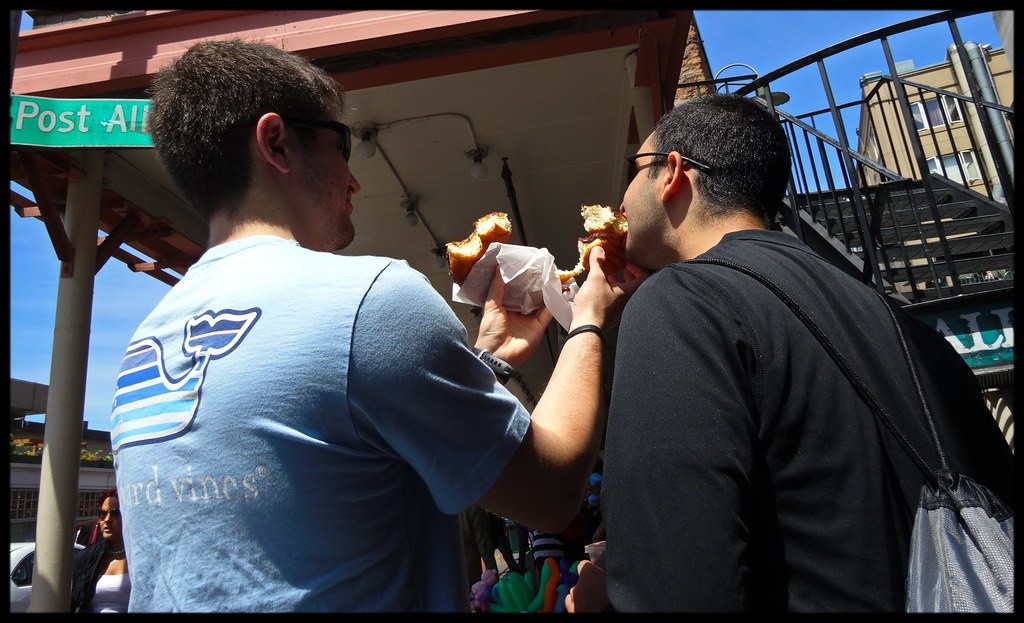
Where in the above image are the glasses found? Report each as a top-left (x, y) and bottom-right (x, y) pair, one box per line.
(280, 114), (352, 165)
(97, 508), (121, 519)
(626, 152), (712, 184)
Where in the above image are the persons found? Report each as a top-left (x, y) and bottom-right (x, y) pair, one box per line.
(65, 486), (130, 613)
(516, 524), (528, 574)
(463, 505), (524, 583)
(109, 38), (664, 613)
(526, 526), (564, 588)
(563, 94), (1015, 615)
(570, 471), (605, 546)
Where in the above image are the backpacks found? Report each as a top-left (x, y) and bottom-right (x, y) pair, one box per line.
(682, 254), (1014, 614)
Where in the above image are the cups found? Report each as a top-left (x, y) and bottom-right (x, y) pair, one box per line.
(584, 541), (606, 573)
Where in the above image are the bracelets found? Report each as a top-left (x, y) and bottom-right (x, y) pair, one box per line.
(563, 323), (603, 345)
(470, 344), (514, 386)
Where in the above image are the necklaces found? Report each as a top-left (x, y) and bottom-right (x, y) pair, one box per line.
(107, 544), (124, 554)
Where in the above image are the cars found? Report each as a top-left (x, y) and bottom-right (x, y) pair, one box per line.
(10, 518), (100, 614)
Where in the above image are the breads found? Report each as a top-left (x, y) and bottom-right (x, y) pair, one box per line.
(447, 204), (628, 287)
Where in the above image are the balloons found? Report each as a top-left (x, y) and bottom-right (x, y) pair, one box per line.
(489, 556), (579, 613)
